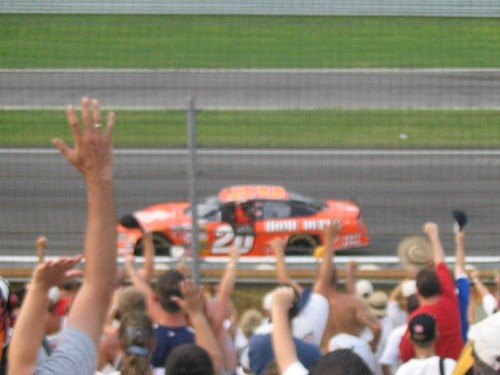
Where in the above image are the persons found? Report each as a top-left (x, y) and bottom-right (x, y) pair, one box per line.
(36, 97), (116, 375)
(456, 231), (500, 375)
(395, 314), (457, 375)
(398, 222), (464, 361)
(239, 217), (418, 375)
(0, 227), (247, 375)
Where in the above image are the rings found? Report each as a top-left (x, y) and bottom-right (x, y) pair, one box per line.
(94, 124), (102, 128)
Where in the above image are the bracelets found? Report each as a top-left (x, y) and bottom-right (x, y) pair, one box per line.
(474, 279), (480, 283)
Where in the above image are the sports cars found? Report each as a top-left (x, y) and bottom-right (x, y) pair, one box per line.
(117, 184), (370, 258)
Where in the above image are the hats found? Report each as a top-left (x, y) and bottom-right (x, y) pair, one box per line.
(114, 288), (147, 318)
(355, 279), (373, 299)
(408, 314), (437, 335)
(453, 210), (467, 234)
(152, 270), (183, 298)
(368, 291), (388, 317)
(399, 236), (433, 274)
